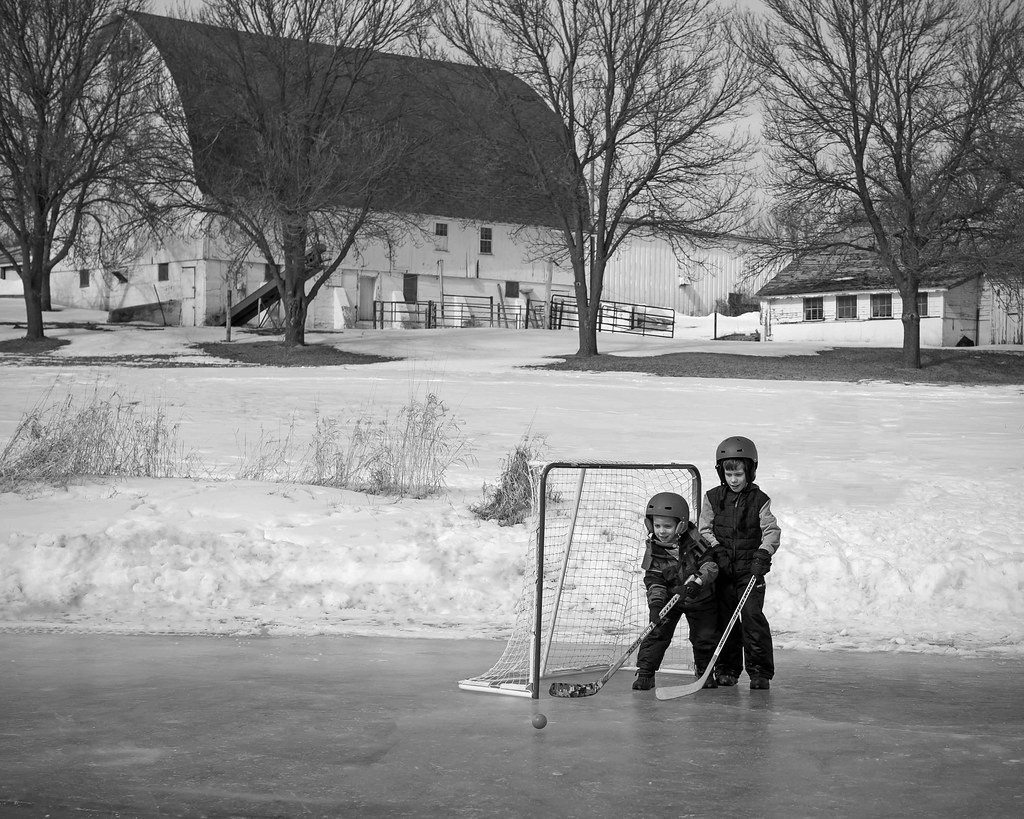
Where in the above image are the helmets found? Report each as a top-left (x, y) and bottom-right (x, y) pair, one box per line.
(644, 492), (690, 535)
(714, 436), (758, 482)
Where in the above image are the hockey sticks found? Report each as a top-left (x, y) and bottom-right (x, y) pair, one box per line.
(549, 573), (697, 697)
(654, 574), (757, 701)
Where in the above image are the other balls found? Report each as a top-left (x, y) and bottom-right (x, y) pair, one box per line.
(532, 714), (547, 729)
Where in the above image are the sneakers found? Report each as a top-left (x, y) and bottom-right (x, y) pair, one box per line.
(632, 669), (655, 689)
(694, 663), (718, 688)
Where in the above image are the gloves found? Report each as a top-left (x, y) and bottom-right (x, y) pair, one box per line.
(648, 602), (669, 629)
(712, 545), (733, 575)
(670, 581), (702, 610)
(750, 552), (769, 585)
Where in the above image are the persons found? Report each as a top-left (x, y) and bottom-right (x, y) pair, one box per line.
(698, 436), (781, 689)
(632, 492), (719, 690)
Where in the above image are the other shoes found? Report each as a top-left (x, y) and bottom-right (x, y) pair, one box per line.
(749, 674), (769, 689)
(715, 671), (738, 686)
